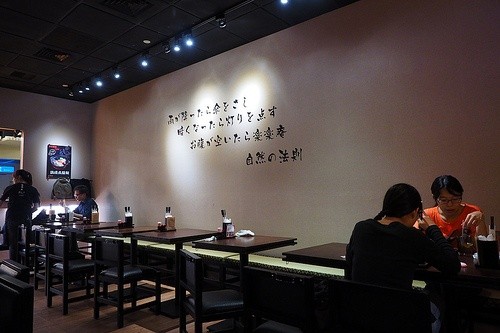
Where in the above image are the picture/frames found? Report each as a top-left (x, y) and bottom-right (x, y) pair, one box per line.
(44, 144), (72, 180)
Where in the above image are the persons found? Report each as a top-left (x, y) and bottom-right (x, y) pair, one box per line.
(343, 183), (461, 333)
(71, 184), (98, 221)
(414, 175), (488, 253)
(0, 169), (40, 250)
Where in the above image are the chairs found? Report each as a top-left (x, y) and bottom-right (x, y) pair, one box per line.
(15, 223), (448, 333)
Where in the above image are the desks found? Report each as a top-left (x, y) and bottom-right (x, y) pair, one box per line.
(90, 226), (159, 312)
(130, 228), (219, 320)
(416, 265), (500, 291)
(190, 234), (298, 333)
(34, 223), (119, 292)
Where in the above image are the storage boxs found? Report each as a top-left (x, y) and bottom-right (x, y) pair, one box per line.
(91, 213), (99, 222)
(83, 225), (100, 228)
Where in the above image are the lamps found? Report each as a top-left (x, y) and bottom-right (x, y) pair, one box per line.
(67, 13), (227, 97)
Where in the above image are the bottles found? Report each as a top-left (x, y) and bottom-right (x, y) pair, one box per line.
(118, 220), (121, 227)
(158, 222), (161, 230)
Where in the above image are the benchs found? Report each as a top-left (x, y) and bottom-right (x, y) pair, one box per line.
(122, 236), (161, 247)
(151, 245), (201, 255)
(226, 254), (348, 281)
(101, 236), (125, 241)
(461, 296), (500, 333)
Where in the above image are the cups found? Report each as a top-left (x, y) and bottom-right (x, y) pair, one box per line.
(49, 210), (55, 220)
(167, 217), (175, 229)
(460, 225), (474, 248)
(477, 239), (499, 269)
(125, 216), (132, 226)
(223, 223), (235, 238)
(92, 212), (99, 222)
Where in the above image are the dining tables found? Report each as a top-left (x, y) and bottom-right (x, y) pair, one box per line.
(280, 242), (349, 269)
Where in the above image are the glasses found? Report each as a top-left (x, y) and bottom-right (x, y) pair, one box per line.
(73, 193), (84, 197)
(436, 198), (462, 204)
